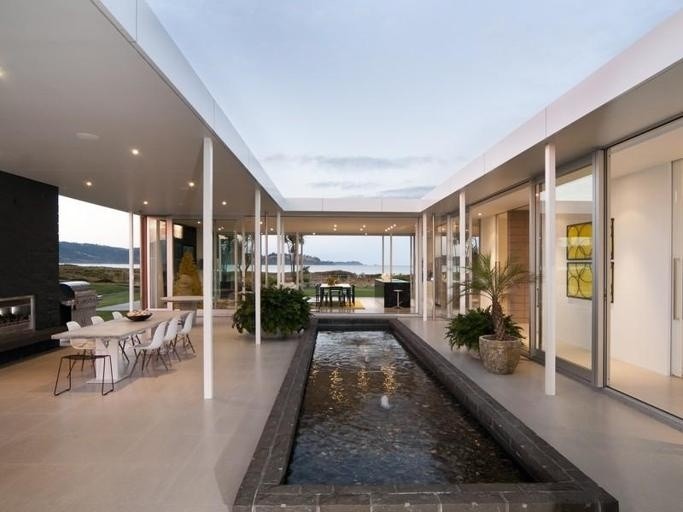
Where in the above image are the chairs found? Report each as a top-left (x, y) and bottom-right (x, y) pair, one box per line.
(315, 282), (356, 306)
(53, 309), (195, 374)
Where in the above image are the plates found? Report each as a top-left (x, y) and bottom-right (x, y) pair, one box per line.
(122, 312), (153, 321)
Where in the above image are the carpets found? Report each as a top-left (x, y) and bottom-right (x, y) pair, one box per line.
(307, 300), (365, 310)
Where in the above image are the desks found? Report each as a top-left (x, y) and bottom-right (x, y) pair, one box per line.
(319, 283), (351, 308)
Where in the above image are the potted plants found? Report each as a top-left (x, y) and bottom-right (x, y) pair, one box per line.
(443, 251), (544, 375)
(230, 285), (314, 340)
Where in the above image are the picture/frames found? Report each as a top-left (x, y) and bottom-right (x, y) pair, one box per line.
(566, 262), (614, 303)
(565, 217), (615, 260)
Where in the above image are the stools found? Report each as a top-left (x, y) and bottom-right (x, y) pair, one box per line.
(53, 354), (115, 397)
(392, 289), (405, 310)
